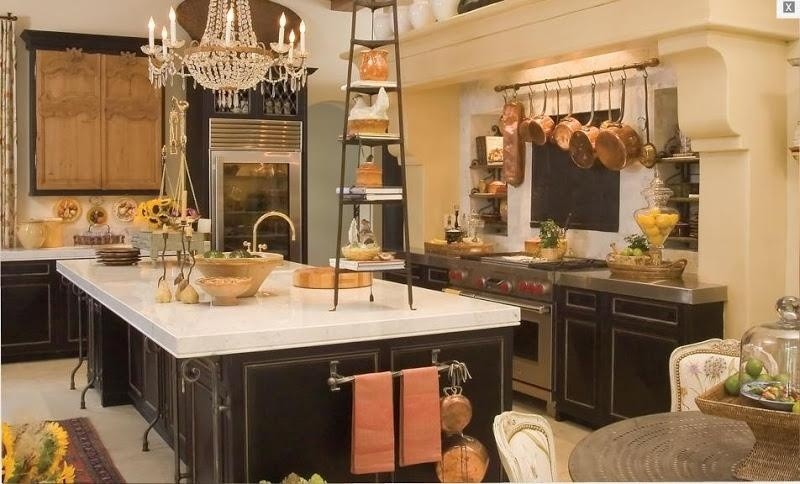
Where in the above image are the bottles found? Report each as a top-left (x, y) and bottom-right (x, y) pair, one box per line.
(479, 180), (486, 194)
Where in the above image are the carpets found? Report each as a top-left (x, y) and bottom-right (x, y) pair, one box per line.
(1, 417), (125, 484)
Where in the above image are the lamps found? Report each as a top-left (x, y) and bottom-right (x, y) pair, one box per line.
(140, 0), (310, 108)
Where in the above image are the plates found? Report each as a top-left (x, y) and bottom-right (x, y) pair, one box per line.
(739, 381), (797, 412)
(94, 248), (141, 268)
(112, 197), (138, 222)
(53, 198), (82, 222)
(86, 205), (109, 227)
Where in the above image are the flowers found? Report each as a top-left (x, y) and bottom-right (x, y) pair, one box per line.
(1, 421), (76, 484)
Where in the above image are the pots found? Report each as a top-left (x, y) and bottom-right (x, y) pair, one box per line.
(432, 434), (491, 482)
(517, 79), (645, 171)
(437, 385), (472, 436)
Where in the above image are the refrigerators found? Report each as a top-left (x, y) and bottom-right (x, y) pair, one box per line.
(208, 118), (304, 263)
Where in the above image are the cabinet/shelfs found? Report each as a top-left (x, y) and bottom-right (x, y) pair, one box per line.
(382, 263), (449, 291)
(556, 285), (723, 427)
(1, 260), (87, 359)
(329, 0), (417, 311)
(655, 154), (699, 242)
(470, 161), (507, 223)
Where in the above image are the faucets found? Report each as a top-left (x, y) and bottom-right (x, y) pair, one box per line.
(250, 210), (296, 252)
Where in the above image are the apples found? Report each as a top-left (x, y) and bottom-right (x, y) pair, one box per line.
(620, 249), (643, 256)
(724, 358), (791, 395)
(793, 399), (800, 413)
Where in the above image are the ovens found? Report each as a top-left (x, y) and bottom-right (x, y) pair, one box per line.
(445, 285), (558, 407)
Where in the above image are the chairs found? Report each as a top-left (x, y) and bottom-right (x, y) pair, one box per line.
(669, 338), (779, 412)
(492, 411), (556, 482)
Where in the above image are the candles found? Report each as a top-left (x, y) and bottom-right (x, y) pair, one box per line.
(163, 224), (168, 233)
(454, 200), (460, 209)
(186, 227), (192, 237)
(181, 189), (188, 221)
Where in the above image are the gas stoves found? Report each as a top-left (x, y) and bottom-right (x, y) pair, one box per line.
(447, 255), (610, 302)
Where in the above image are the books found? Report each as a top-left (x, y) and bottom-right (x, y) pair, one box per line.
(329, 258), (405, 272)
(336, 185), (403, 203)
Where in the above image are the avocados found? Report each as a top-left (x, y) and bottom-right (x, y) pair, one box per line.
(204, 250), (262, 259)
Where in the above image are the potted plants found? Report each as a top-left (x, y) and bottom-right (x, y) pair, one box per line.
(538, 218), (563, 260)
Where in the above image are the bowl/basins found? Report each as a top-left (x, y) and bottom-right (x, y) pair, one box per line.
(190, 252), (287, 302)
(340, 245), (382, 263)
(348, 118), (389, 135)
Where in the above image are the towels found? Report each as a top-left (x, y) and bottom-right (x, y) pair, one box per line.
(399, 366), (442, 468)
(351, 371), (395, 475)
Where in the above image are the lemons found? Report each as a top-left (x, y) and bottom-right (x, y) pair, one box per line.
(635, 213), (679, 246)
